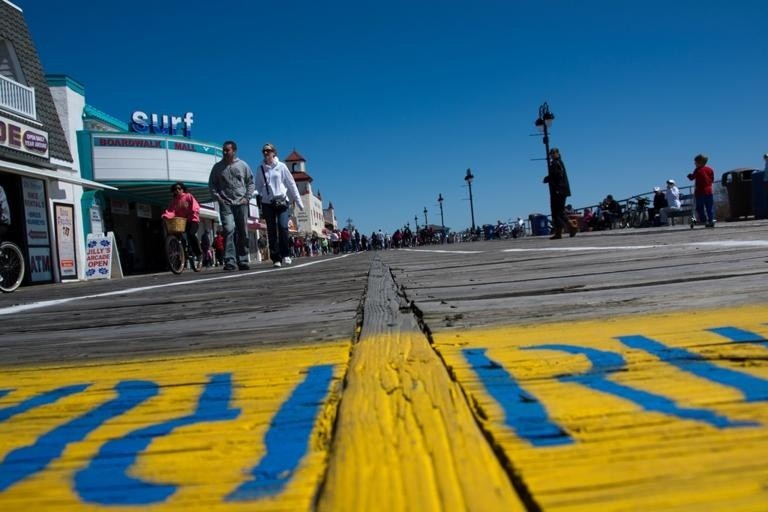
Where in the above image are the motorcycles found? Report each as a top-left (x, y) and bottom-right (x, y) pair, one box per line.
(563, 210), (584, 236)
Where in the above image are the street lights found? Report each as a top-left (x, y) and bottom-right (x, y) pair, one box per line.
(401, 222), (410, 230)
(413, 214), (418, 228)
(423, 206), (428, 227)
(459, 167), (476, 230)
(530, 102), (554, 170)
(438, 193), (444, 226)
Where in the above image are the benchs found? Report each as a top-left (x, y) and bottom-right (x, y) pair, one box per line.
(652, 192), (695, 227)
(612, 204), (627, 229)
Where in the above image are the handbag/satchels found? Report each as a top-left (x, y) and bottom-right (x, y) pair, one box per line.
(270, 195), (289, 212)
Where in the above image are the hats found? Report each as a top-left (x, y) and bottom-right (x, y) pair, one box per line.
(653, 187), (660, 192)
(667, 179), (674, 184)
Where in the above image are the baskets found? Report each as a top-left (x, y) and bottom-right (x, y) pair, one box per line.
(165, 215), (188, 234)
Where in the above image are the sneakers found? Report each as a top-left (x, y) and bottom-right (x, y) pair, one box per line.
(284, 256), (292, 264)
(238, 264), (251, 270)
(569, 227), (578, 237)
(272, 261), (282, 268)
(222, 264), (235, 271)
(549, 234), (562, 240)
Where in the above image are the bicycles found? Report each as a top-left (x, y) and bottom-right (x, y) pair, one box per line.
(619, 194), (651, 229)
(163, 215), (203, 275)
(0, 241), (25, 294)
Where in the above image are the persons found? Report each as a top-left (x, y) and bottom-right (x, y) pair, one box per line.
(256, 235), (267, 261)
(254, 142), (303, 268)
(125, 234), (137, 275)
(205, 140), (255, 272)
(577, 179), (683, 233)
(199, 225), (226, 268)
(686, 154), (715, 227)
(158, 181), (201, 259)
(287, 217), (527, 257)
(540, 147), (577, 240)
(0, 184), (12, 283)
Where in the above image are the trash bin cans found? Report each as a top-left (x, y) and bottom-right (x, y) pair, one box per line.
(528, 214), (548, 236)
(721, 168), (759, 221)
(482, 224), (494, 240)
